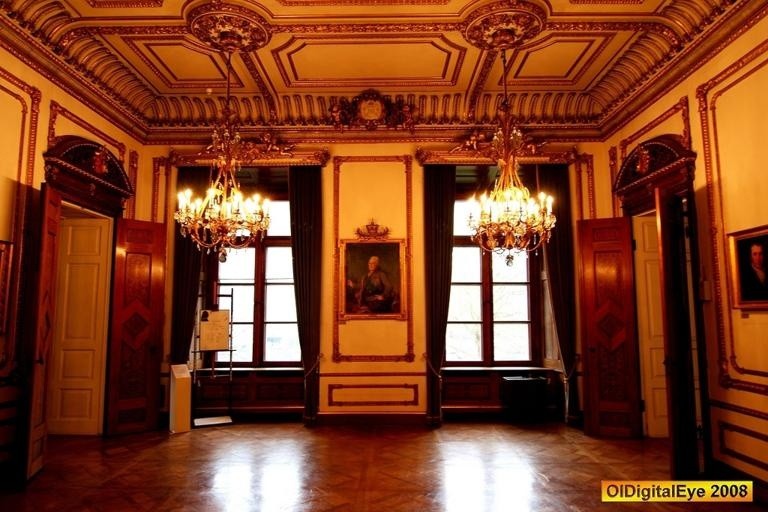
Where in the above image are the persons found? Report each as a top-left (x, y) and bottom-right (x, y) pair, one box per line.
(348, 255), (394, 314)
(741, 237), (767, 300)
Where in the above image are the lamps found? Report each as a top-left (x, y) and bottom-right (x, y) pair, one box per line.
(174, 34), (274, 265)
(466, 32), (554, 268)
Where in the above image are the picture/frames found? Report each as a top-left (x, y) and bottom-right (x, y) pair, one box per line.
(340, 239), (409, 323)
(725, 224), (768, 312)
